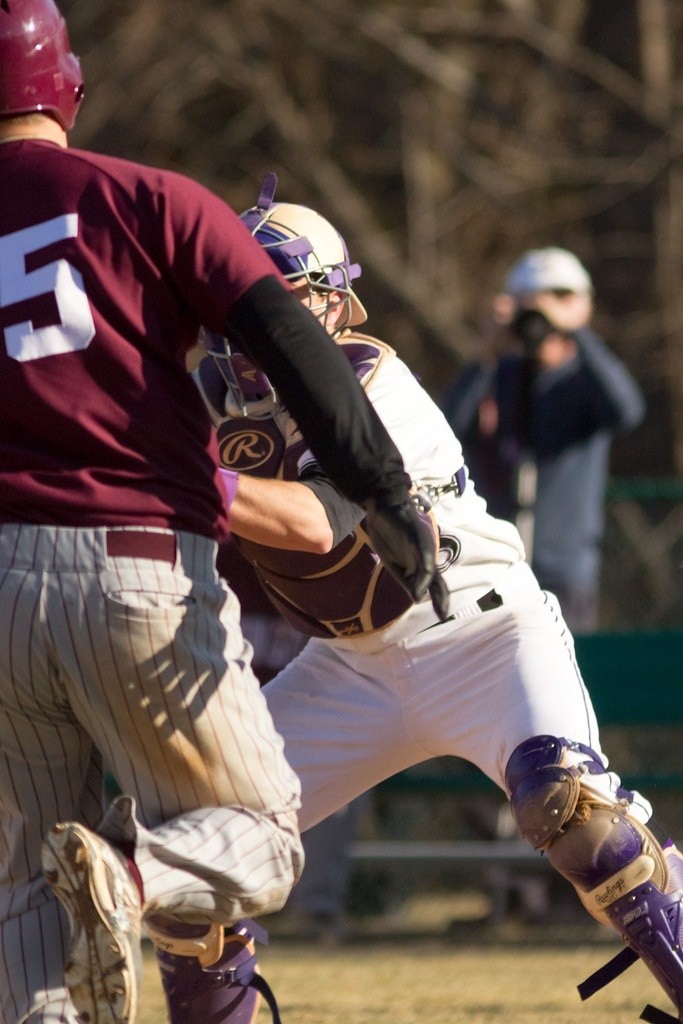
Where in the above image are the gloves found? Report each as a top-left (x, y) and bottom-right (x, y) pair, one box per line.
(367, 487), (452, 620)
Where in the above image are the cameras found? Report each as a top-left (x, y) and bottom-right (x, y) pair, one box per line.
(507, 308), (554, 350)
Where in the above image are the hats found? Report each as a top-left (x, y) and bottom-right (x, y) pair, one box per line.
(502, 247), (593, 293)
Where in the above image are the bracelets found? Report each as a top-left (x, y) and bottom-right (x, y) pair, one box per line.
(213, 465), (238, 511)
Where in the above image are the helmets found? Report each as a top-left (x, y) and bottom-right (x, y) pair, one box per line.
(0, 0), (86, 130)
(203, 176), (370, 326)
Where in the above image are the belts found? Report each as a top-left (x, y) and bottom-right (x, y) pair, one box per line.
(422, 589), (505, 635)
(106, 530), (178, 564)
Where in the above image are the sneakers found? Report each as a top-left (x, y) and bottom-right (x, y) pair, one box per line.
(38, 820), (144, 1024)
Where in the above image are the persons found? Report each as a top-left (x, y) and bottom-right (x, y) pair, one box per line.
(1, 0), (451, 1024)
(145, 170), (683, 1024)
